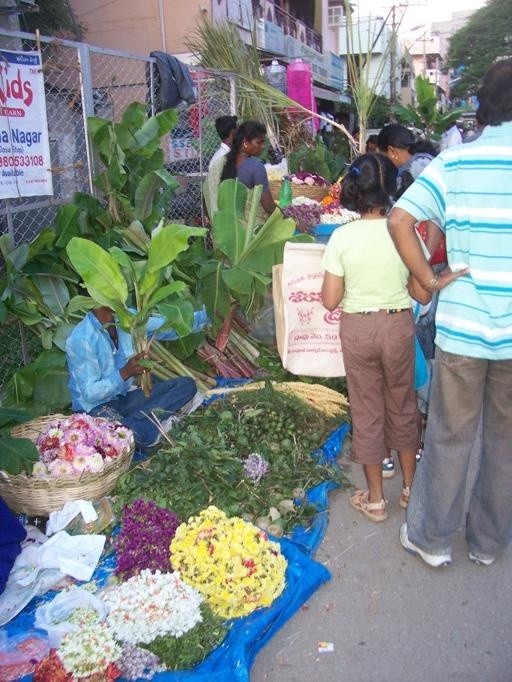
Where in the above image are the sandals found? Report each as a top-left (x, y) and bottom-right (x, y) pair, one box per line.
(399, 487), (411, 508)
(349, 489), (390, 522)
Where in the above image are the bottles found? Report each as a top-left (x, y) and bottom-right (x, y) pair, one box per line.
(278, 176), (293, 208)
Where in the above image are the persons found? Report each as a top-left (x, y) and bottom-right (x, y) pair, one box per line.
(373, 120), (439, 181)
(63, 276), (237, 463)
(378, 296), (434, 480)
(310, 103), (488, 159)
(380, 52), (510, 569)
(201, 114), (241, 236)
(411, 216), (448, 428)
(320, 151), (432, 524)
(219, 120), (294, 240)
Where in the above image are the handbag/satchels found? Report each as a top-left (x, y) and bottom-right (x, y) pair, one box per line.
(272, 242), (346, 378)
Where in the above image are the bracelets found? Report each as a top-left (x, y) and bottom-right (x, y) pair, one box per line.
(429, 271), (443, 288)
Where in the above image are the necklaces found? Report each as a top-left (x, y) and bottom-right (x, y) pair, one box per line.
(238, 151), (250, 157)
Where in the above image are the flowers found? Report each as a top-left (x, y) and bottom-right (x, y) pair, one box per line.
(280, 173), (358, 233)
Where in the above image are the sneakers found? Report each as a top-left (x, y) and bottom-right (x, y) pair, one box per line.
(382, 457), (396, 479)
(400, 523), (452, 568)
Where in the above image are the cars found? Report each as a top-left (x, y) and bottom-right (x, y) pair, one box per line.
(163, 156), (212, 248)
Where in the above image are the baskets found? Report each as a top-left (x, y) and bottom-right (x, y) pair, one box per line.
(269, 179), (330, 204)
(1, 414), (136, 521)
